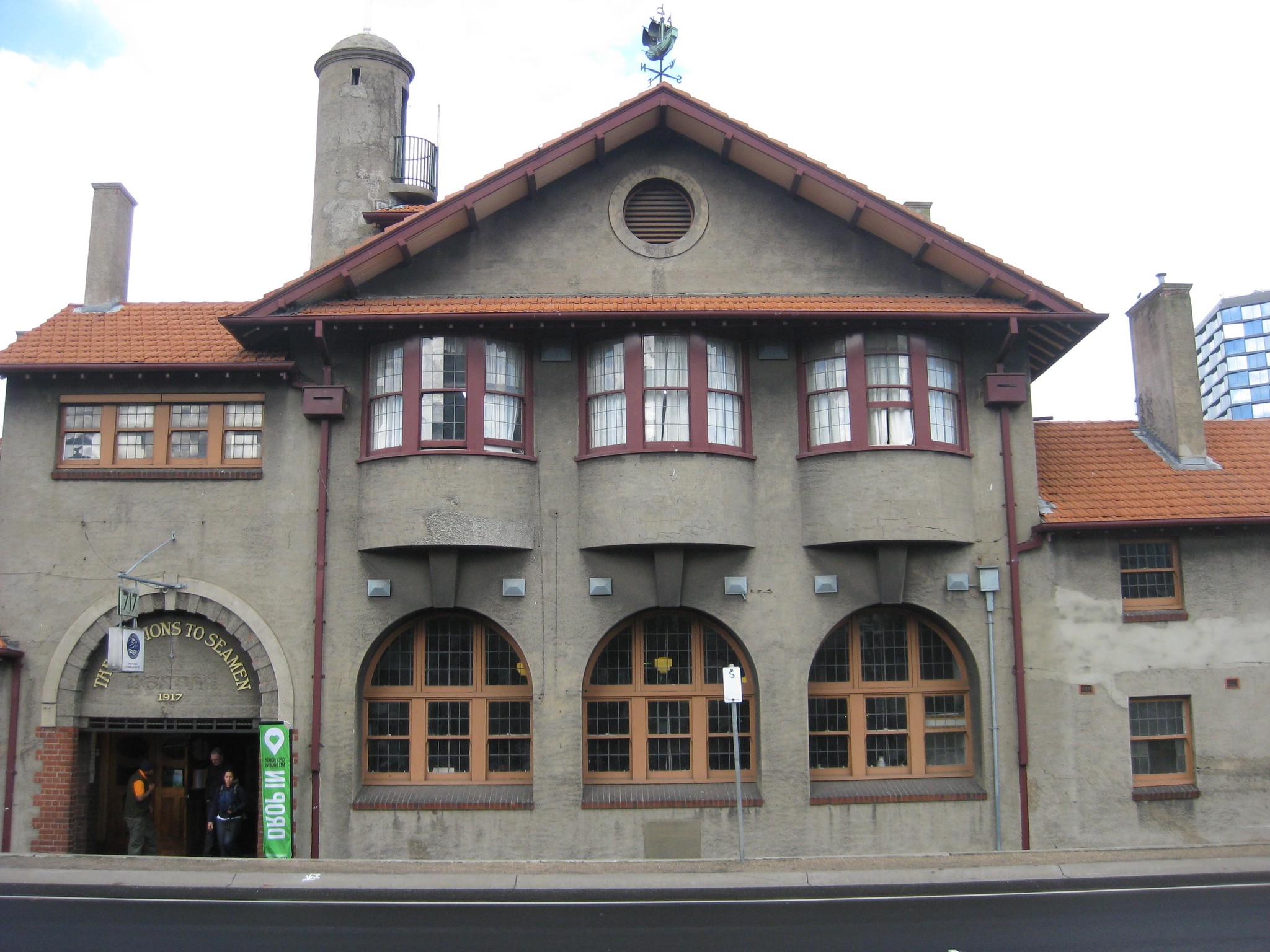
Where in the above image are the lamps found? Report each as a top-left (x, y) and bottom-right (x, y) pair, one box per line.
(814, 575), (838, 593)
(503, 579), (525, 596)
(654, 657), (672, 674)
(590, 577), (612, 595)
(368, 579), (391, 596)
(724, 577), (748, 600)
(517, 661), (526, 676)
(947, 572), (969, 590)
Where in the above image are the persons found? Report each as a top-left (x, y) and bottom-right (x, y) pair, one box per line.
(124, 758), (161, 856)
(203, 748), (248, 857)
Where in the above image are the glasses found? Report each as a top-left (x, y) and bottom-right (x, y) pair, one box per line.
(211, 758), (218, 762)
